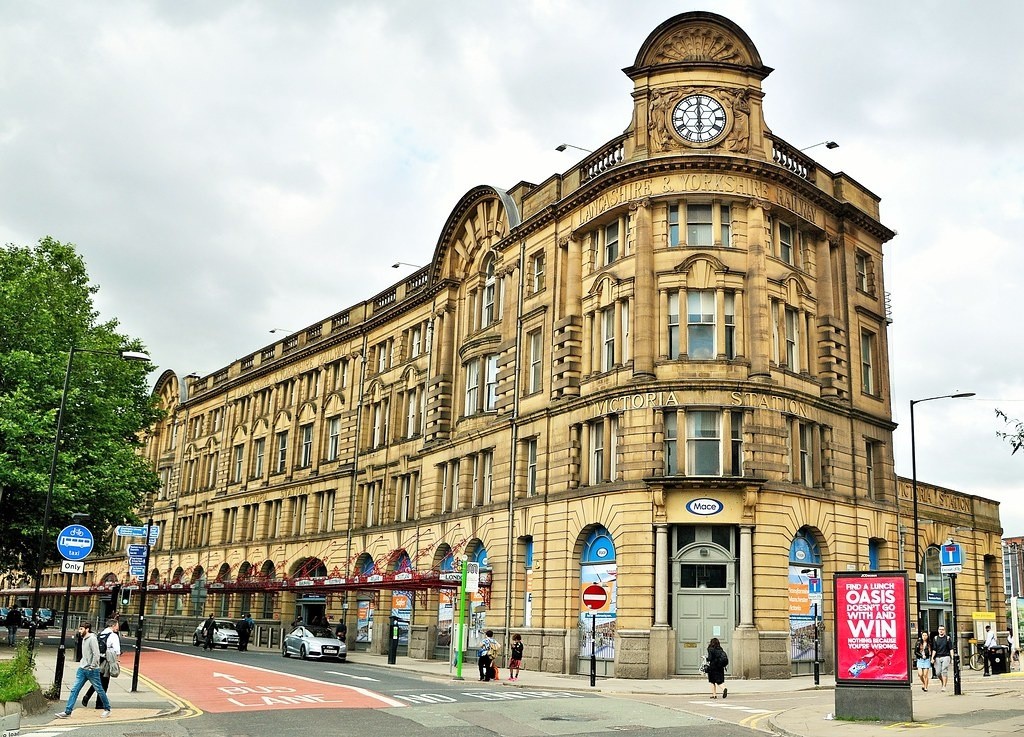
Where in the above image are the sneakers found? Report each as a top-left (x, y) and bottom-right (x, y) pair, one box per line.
(101, 710), (110, 717)
(55, 713), (71, 719)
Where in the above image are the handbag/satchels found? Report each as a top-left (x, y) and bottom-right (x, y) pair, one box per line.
(699, 657), (709, 673)
(490, 664), (498, 680)
(203, 627), (207, 638)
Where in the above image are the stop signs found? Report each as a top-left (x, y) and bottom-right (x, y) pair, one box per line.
(582, 584), (607, 610)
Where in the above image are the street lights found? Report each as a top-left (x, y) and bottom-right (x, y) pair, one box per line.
(801, 569), (819, 685)
(28, 347), (150, 665)
(910, 393), (976, 638)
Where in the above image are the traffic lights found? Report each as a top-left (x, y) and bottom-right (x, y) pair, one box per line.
(122, 589), (130, 605)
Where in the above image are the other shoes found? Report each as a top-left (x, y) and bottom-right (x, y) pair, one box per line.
(478, 678), (483, 681)
(507, 678), (513, 681)
(924, 689), (928, 691)
(922, 687), (925, 690)
(95, 705), (103, 709)
(941, 687), (946, 692)
(710, 695), (717, 699)
(722, 688), (727, 698)
(482, 679), (489, 682)
(82, 695), (88, 707)
(512, 678), (518, 681)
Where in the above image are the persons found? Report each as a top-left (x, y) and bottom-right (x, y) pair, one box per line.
(478, 629), (500, 681)
(914, 630), (933, 691)
(5, 604), (22, 647)
(201, 613), (218, 652)
(245, 613), (255, 651)
(81, 618), (121, 709)
(983, 625), (997, 651)
(55, 621), (112, 719)
(700, 638), (727, 699)
(508, 634), (524, 680)
(1007, 628), (1016, 668)
(930, 625), (953, 692)
(291, 615), (347, 643)
(236, 614), (251, 652)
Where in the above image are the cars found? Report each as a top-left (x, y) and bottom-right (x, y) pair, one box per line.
(192, 620), (239, 649)
(40, 609), (54, 626)
(282, 625), (347, 662)
(20, 608), (47, 628)
(0, 608), (10, 625)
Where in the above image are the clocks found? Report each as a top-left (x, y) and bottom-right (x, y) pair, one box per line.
(672, 94), (726, 143)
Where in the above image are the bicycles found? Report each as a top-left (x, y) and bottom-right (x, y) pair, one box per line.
(969, 644), (991, 671)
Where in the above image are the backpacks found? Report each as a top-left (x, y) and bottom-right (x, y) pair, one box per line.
(96, 630), (111, 665)
(486, 638), (499, 660)
(712, 646), (728, 671)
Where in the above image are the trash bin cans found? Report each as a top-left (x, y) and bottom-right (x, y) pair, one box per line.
(73, 631), (93, 662)
(990, 645), (1010, 675)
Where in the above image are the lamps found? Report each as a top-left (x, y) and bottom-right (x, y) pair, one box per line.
(392, 262), (422, 268)
(555, 143), (594, 154)
(798, 141), (839, 151)
(269, 328), (295, 333)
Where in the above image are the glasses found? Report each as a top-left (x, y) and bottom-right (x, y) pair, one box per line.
(939, 629), (944, 631)
